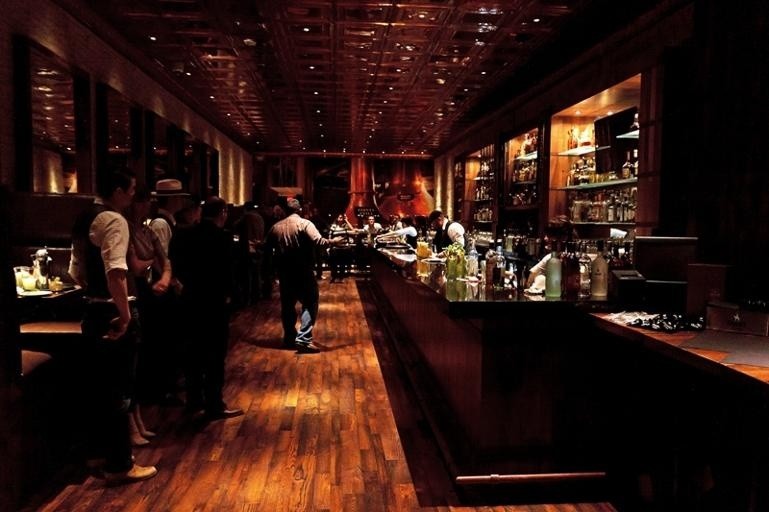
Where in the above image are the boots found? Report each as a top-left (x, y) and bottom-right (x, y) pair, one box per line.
(131, 404), (157, 439)
(128, 412), (150, 447)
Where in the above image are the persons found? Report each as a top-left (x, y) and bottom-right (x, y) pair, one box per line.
(69, 168), (467, 486)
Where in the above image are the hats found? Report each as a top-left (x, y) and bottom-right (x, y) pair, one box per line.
(150, 178), (191, 197)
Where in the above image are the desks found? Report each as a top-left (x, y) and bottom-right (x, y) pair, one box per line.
(589, 309), (769, 400)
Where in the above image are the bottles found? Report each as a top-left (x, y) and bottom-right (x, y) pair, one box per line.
(465, 238), (505, 288)
(544, 227), (632, 300)
(506, 133), (536, 256)
(566, 150), (639, 223)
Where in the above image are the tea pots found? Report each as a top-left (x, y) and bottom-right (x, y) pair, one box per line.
(29, 249), (52, 289)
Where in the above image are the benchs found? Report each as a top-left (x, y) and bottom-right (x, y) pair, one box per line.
(1, 206), (53, 511)
(19, 245), (83, 355)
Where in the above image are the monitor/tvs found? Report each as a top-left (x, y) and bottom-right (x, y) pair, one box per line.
(633, 236), (699, 278)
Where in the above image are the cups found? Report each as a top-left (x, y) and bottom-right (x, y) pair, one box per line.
(416, 238), (429, 260)
(416, 260), (431, 277)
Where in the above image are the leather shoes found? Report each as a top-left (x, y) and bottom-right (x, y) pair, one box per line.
(294, 342), (319, 354)
(105, 463), (156, 486)
(204, 407), (243, 417)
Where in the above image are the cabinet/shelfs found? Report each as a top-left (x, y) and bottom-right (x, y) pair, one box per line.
(455, 33), (673, 267)
(12, 31), (220, 196)
